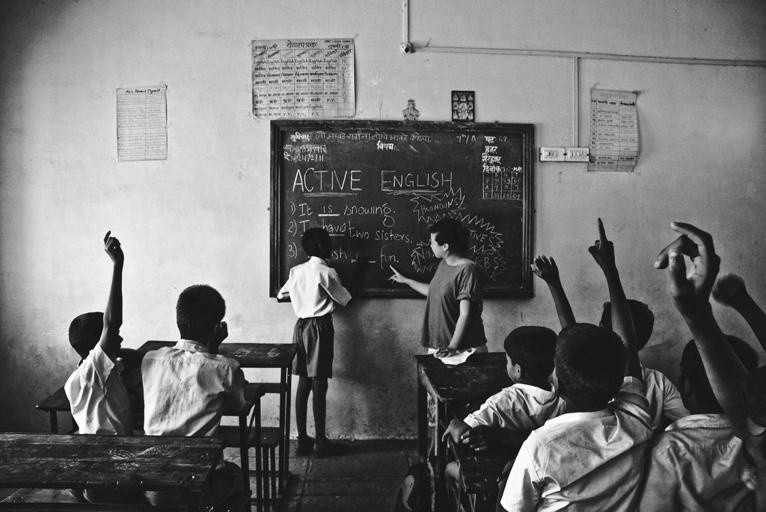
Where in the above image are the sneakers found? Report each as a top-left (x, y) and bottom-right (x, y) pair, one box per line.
(296, 436), (316, 455)
(313, 438), (341, 458)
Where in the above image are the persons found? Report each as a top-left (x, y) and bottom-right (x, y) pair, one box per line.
(386, 219), (490, 459)
(275, 227), (354, 460)
(139, 283), (258, 511)
(392, 214), (766, 511)
(61, 229), (148, 507)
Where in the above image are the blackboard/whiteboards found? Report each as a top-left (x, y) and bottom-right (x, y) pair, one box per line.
(268, 118), (536, 301)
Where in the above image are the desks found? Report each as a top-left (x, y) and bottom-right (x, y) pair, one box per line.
(412, 347), (555, 512)
